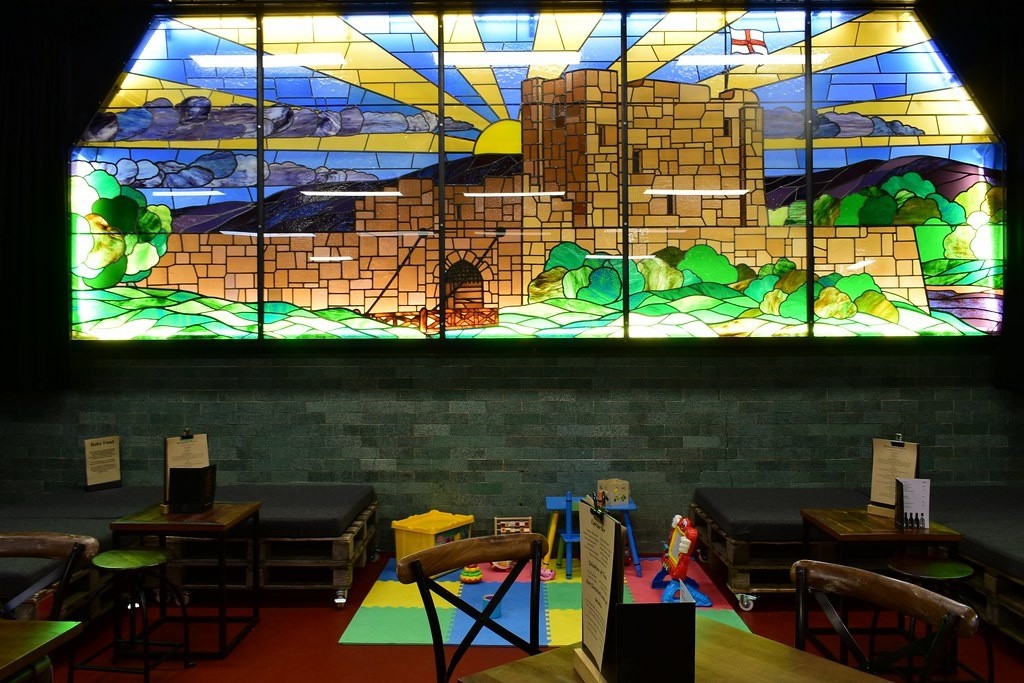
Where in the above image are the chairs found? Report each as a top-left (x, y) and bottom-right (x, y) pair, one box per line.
(393, 533), (549, 683)
(788, 560), (979, 683)
(556, 492), (581, 579)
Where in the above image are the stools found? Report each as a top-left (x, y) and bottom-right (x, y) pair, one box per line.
(65, 550), (195, 683)
(867, 555), (995, 683)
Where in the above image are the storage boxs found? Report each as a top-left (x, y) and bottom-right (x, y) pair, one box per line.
(389, 510), (474, 578)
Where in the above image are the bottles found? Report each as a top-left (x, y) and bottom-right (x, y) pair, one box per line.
(904, 512), (925, 528)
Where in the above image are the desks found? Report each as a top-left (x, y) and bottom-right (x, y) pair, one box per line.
(459, 614), (891, 683)
(541, 497), (643, 577)
(801, 507), (961, 665)
(0, 621), (83, 683)
(110, 503), (264, 660)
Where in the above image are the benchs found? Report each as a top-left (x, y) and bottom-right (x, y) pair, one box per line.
(0, 481), (378, 622)
(695, 486), (1024, 668)
